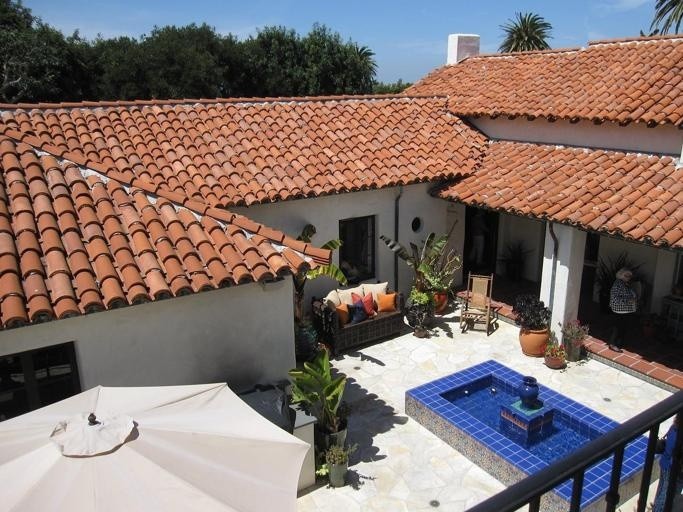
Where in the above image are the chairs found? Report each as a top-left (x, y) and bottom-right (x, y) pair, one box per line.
(458, 271), (503, 336)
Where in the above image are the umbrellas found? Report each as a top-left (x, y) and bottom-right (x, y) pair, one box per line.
(0, 383), (311, 512)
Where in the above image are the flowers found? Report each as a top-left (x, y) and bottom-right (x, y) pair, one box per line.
(539, 330), (571, 368)
(556, 318), (591, 348)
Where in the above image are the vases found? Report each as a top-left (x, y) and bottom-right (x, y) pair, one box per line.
(561, 333), (583, 363)
(518, 376), (540, 409)
(542, 354), (565, 369)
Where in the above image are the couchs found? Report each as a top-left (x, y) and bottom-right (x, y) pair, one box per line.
(309, 284), (406, 357)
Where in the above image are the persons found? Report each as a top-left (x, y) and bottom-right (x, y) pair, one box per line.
(652, 415), (683, 512)
(340, 256), (358, 284)
(609, 268), (636, 352)
(470, 210), (489, 265)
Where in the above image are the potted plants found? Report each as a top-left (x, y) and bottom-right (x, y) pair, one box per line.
(285, 350), (349, 451)
(588, 249), (648, 314)
(322, 440), (362, 488)
(430, 218), (465, 314)
(376, 229), (458, 339)
(508, 290), (555, 360)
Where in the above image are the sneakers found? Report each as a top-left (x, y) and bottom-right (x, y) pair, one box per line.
(609, 343), (624, 354)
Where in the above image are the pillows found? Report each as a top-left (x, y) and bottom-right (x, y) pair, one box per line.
(322, 281), (396, 326)
